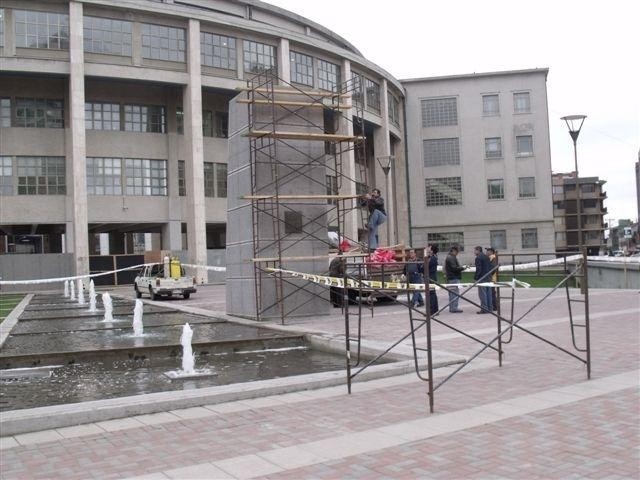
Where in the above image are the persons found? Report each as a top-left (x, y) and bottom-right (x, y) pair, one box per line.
(364, 188), (388, 251)
(473, 246), (495, 314)
(445, 245), (468, 313)
(403, 247), (424, 307)
(485, 247), (499, 310)
(421, 244), (440, 316)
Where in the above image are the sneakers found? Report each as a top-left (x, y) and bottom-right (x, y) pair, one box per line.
(449, 307), (497, 314)
(417, 303), (424, 307)
(364, 224), (372, 230)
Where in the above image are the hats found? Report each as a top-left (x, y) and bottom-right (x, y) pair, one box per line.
(485, 246), (493, 250)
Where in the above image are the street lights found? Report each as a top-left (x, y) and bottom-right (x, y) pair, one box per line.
(377, 154), (396, 247)
(560, 115), (588, 253)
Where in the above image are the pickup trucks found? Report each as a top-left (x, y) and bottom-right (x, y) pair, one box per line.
(133, 262), (197, 300)
(329, 254), (400, 308)
